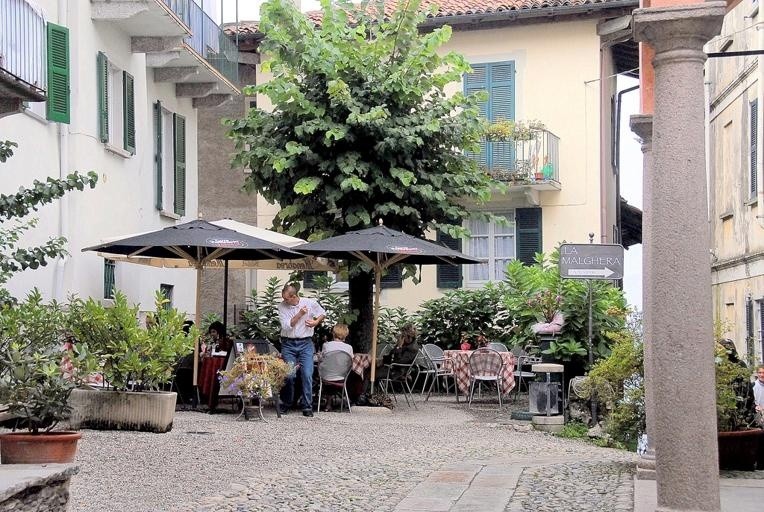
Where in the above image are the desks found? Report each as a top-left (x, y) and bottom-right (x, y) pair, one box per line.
(196, 349), (384, 411)
(440, 348), (517, 404)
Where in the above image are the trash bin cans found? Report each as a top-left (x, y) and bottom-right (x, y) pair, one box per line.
(539, 333), (584, 414)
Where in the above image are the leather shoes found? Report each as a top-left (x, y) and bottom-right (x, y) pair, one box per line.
(304, 411), (313, 417)
(280, 407), (287, 415)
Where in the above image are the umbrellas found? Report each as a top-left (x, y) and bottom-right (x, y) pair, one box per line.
(80, 216), (307, 406)
(96, 217), (340, 340)
(289, 224), (488, 394)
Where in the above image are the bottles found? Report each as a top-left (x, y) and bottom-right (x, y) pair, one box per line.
(200, 340), (219, 354)
(305, 308), (312, 322)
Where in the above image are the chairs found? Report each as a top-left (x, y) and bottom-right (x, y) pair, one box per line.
(315, 348), (355, 416)
(478, 342), (510, 401)
(465, 346), (503, 411)
(375, 347), (418, 412)
(408, 343), (449, 398)
(421, 343), (460, 405)
(167, 337), (419, 419)
(509, 344), (544, 406)
(365, 343), (386, 358)
(230, 338), (283, 419)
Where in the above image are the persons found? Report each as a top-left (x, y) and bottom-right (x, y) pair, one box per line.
(752, 365), (764, 418)
(145, 313), (160, 330)
(355, 322), (420, 406)
(277, 284), (327, 417)
(316, 323), (354, 413)
(199, 321), (234, 357)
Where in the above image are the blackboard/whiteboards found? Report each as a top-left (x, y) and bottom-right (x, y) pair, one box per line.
(234, 341), (280, 401)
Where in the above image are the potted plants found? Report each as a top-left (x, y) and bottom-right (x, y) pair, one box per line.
(713, 317), (763, 471)
(0, 285), (84, 464)
(64, 282), (201, 432)
